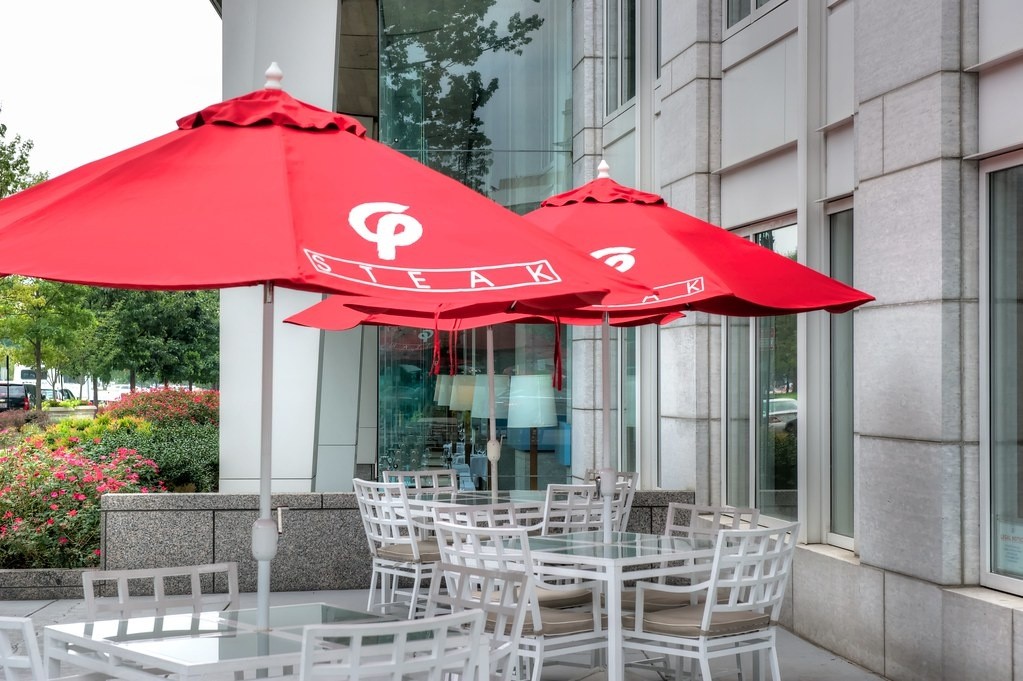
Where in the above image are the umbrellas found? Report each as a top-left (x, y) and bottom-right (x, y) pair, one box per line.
(284, 158), (877, 613)
(0, 61), (687, 681)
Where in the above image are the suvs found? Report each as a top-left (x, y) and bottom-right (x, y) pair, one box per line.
(0, 381), (46, 412)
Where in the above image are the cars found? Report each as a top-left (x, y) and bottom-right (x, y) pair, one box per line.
(763, 398), (798, 431)
(13, 362), (198, 401)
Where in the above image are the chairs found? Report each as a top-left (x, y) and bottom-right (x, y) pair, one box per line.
(0, 616), (100, 681)
(82, 562), (240, 603)
(301, 468), (803, 681)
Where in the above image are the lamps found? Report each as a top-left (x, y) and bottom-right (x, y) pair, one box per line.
(434, 375), (438, 401)
(450, 374), (471, 411)
(471, 373), (507, 488)
(508, 373), (558, 488)
(439, 373), (450, 406)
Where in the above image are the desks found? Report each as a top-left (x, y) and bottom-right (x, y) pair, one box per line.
(448, 532), (760, 681)
(44, 603), (434, 680)
(385, 489), (597, 528)
